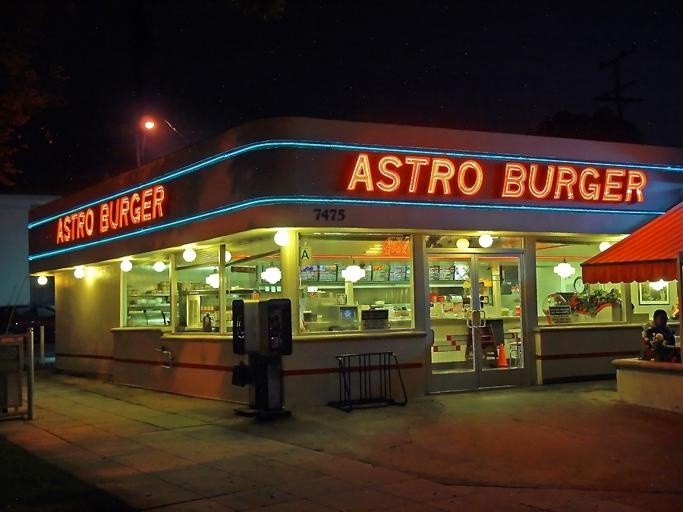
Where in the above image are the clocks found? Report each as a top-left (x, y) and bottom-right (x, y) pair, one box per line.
(573, 277), (586, 295)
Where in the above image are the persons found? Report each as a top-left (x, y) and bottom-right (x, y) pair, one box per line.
(642, 309), (675, 345)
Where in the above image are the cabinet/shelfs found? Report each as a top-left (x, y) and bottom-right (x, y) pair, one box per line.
(127, 288), (253, 328)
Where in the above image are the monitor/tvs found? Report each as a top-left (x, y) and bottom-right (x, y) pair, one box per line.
(339, 305), (359, 323)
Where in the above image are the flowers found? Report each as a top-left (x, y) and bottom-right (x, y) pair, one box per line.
(640, 330), (664, 361)
(570, 290), (620, 316)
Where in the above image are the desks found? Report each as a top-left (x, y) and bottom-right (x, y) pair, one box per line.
(301, 316), (548, 367)
(663, 342), (680, 361)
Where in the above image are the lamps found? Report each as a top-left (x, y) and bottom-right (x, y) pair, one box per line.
(552, 258), (575, 278)
(456, 233), (495, 249)
(35, 230), (295, 289)
(338, 260), (368, 285)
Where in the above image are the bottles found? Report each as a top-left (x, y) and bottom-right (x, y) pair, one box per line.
(500, 308), (509, 316)
(515, 306), (520, 316)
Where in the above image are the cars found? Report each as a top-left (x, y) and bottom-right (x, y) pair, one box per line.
(0, 304), (54, 343)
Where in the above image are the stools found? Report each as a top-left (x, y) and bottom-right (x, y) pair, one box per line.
(464, 322), (500, 365)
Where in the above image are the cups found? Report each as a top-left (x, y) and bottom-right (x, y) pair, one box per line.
(437, 297), (443, 302)
(674, 335), (680, 346)
(394, 311), (400, 317)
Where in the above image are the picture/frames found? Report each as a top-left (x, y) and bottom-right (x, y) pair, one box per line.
(638, 282), (670, 306)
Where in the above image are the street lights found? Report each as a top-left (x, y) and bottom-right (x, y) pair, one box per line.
(133, 116), (156, 167)
